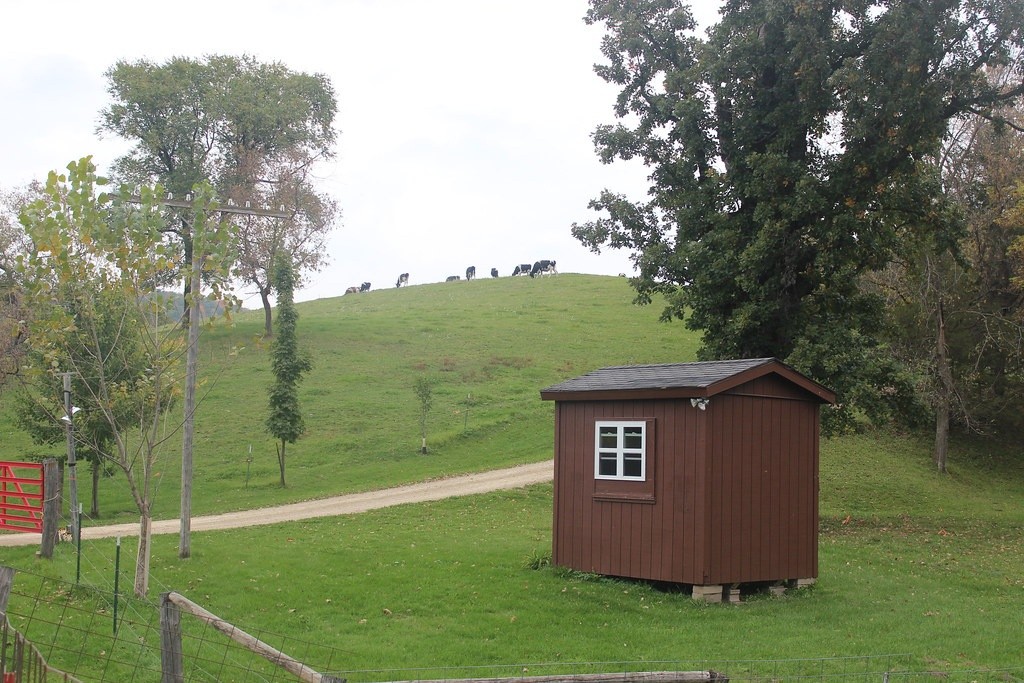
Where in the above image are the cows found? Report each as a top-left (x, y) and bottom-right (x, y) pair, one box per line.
(345, 287), (360, 293)
(529, 260), (559, 279)
(466, 266), (475, 281)
(445, 276), (460, 283)
(396, 273), (409, 288)
(512, 264), (531, 277)
(360, 282), (371, 292)
